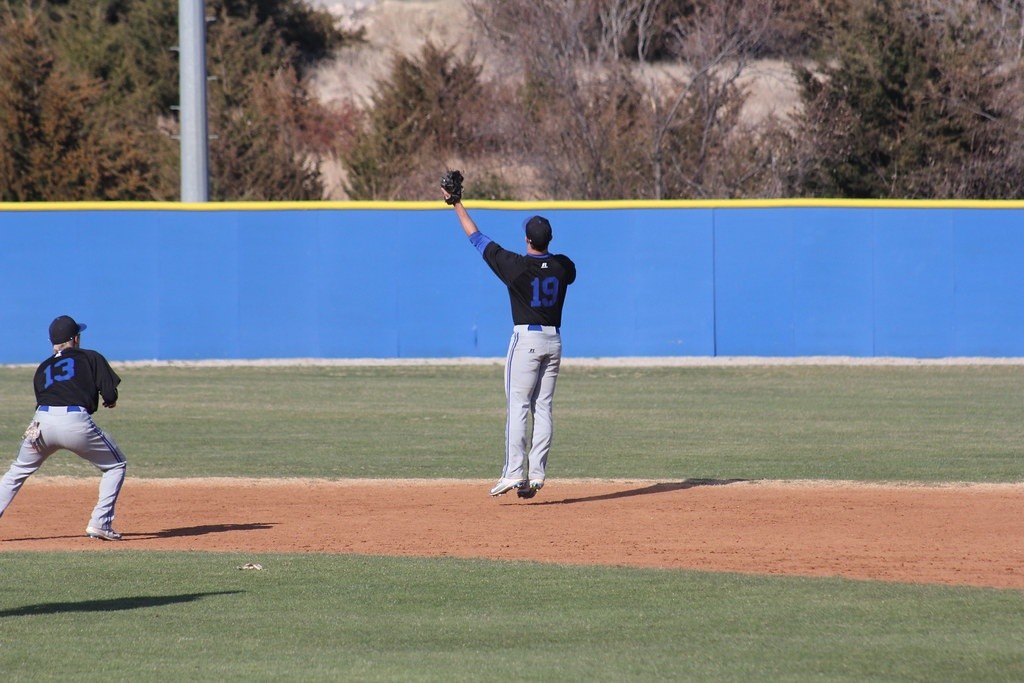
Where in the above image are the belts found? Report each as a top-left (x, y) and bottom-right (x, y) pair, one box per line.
(515, 324), (561, 334)
(37, 405), (81, 412)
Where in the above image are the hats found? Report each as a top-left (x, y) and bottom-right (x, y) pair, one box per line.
(48, 315), (87, 344)
(521, 215), (553, 252)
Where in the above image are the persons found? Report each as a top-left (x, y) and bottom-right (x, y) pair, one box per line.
(440, 170), (576, 499)
(0, 316), (127, 541)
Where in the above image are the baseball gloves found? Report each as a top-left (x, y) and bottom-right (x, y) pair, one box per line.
(440, 170), (465, 206)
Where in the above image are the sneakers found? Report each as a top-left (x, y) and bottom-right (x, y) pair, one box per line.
(517, 479), (544, 499)
(85, 526), (122, 540)
(489, 475), (527, 498)
